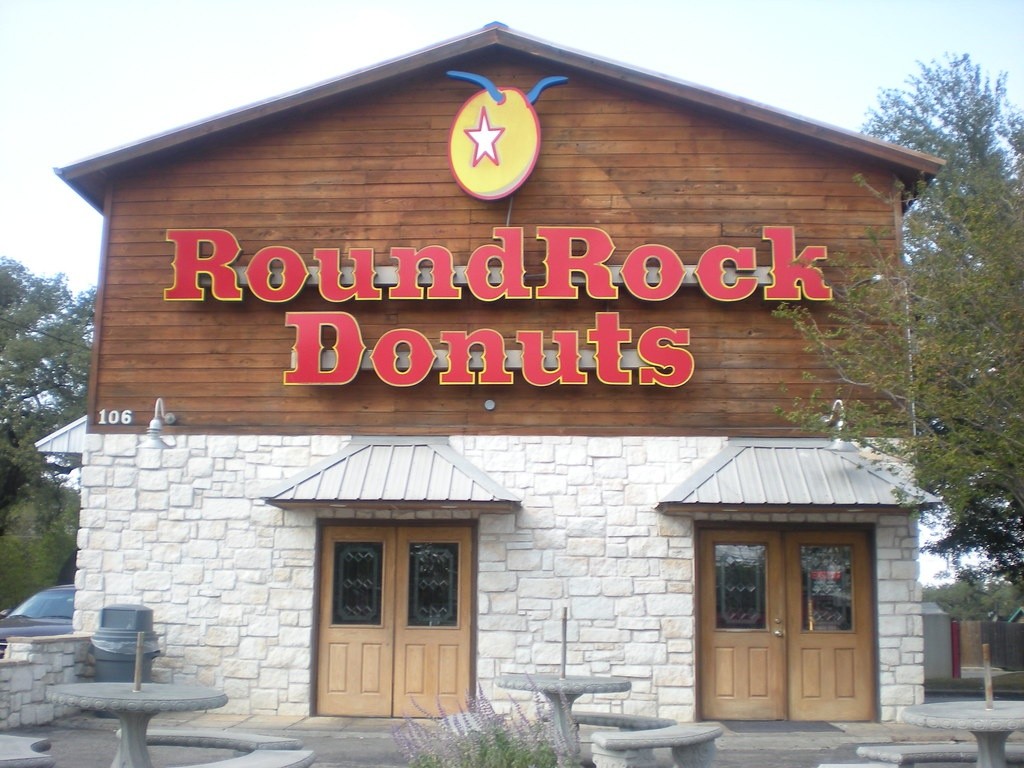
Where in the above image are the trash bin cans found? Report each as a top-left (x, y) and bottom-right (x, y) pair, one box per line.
(88, 604), (160, 719)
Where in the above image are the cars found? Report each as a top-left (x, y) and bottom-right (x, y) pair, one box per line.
(0, 585), (75, 660)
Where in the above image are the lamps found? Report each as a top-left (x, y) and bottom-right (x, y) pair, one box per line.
(821, 400), (862, 453)
(136, 398), (176, 449)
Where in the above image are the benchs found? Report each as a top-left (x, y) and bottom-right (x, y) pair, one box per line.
(857, 743), (1024, 768)
(0, 728), (317, 768)
(536, 710), (724, 768)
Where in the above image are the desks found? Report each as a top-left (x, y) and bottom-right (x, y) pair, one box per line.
(45, 682), (229, 768)
(494, 673), (632, 768)
(898, 700), (1024, 768)
